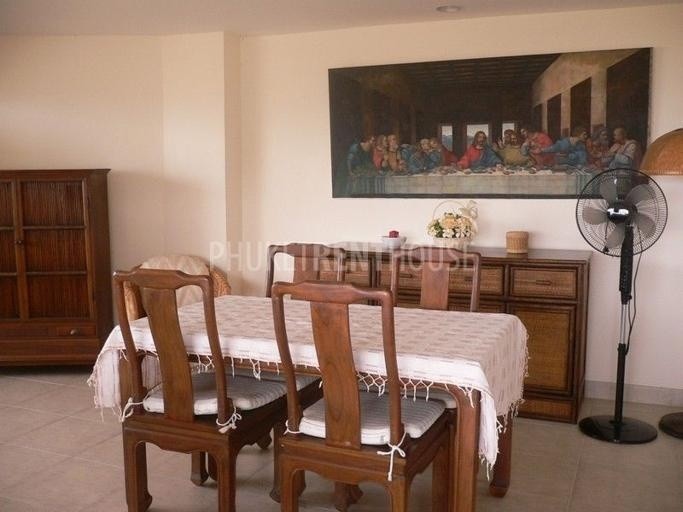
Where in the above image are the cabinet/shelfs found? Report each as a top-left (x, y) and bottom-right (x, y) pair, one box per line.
(0, 167), (115, 375)
(279, 239), (594, 428)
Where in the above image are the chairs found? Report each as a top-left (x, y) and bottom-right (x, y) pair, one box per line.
(348, 245), (483, 499)
(267, 278), (452, 512)
(108, 255), (233, 325)
(204, 241), (345, 493)
(114, 268), (295, 510)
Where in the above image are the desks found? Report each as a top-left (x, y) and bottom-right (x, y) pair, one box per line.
(114, 292), (521, 512)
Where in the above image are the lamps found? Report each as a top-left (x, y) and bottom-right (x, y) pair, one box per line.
(634, 126), (681, 445)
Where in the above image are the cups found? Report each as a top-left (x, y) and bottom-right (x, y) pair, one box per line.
(506, 230), (530, 254)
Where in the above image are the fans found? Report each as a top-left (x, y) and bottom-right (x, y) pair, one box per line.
(571, 164), (667, 448)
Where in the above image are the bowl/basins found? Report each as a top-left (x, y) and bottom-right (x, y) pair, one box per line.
(380, 235), (408, 249)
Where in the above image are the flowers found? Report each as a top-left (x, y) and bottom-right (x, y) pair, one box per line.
(424, 197), (483, 240)
